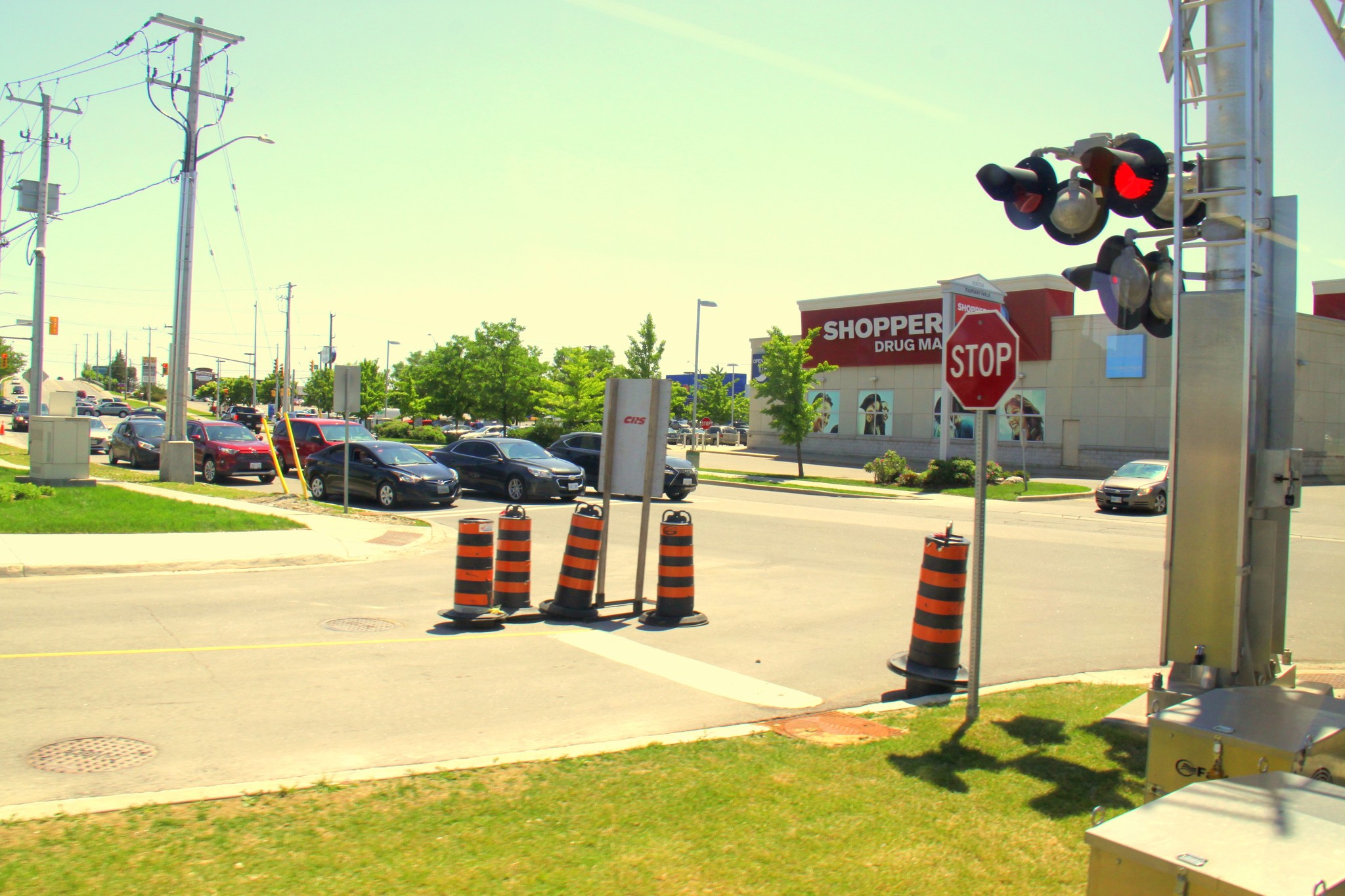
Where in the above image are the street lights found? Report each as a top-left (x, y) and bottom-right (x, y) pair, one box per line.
(216, 360), (225, 378)
(691, 299), (718, 450)
(385, 340), (401, 418)
(427, 333), (438, 350)
(244, 353), (255, 378)
(687, 360), (701, 429)
(726, 363), (738, 427)
(164, 133), (276, 441)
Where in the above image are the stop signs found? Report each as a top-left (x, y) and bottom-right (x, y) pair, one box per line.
(701, 417), (711, 429)
(943, 309), (1020, 411)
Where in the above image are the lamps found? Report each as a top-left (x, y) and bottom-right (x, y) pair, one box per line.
(1296, 358), (1308, 367)
(1018, 373), (1026, 379)
(870, 377), (879, 381)
(818, 378), (827, 382)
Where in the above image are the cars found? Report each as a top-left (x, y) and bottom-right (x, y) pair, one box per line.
(428, 438), (587, 503)
(1095, 458), (1170, 514)
(302, 440), (462, 510)
(669, 419), (749, 446)
(402, 418), (537, 441)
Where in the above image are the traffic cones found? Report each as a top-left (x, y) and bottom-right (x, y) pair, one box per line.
(0, 421), (5, 435)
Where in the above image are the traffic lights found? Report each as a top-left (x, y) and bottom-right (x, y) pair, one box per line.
(162, 363), (169, 375)
(1, 354), (7, 368)
(1062, 234), (1151, 329)
(271, 387), (290, 397)
(274, 359), (284, 378)
(309, 360), (318, 373)
(974, 139), (1169, 230)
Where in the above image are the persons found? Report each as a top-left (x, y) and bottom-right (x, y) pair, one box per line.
(809, 393), (838, 433)
(89, 376), (91, 383)
(354, 450), (371, 464)
(276, 409), (283, 421)
(857, 394), (889, 436)
(140, 392), (144, 400)
(1004, 394), (1044, 441)
(126, 427), (133, 436)
(933, 395), (972, 438)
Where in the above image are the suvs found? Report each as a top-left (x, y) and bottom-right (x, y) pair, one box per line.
(545, 431), (699, 501)
(0, 377), (379, 484)
